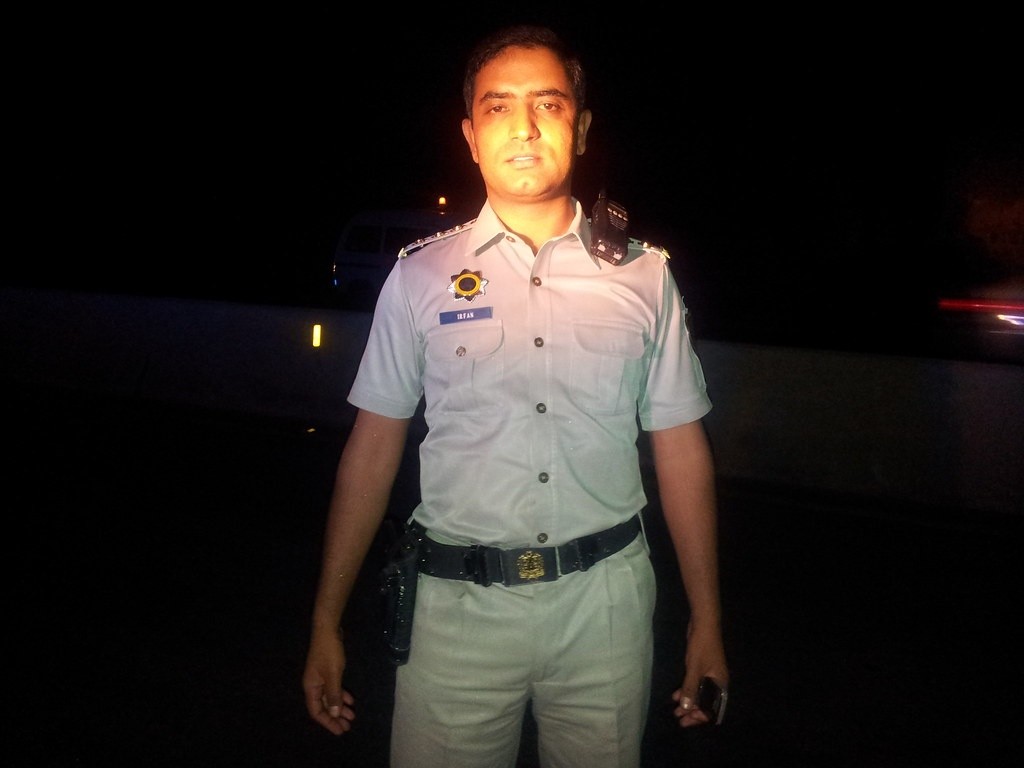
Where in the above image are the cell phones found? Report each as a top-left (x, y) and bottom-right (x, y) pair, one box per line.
(695, 676), (728, 724)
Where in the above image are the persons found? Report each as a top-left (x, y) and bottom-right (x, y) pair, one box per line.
(305, 26), (728, 768)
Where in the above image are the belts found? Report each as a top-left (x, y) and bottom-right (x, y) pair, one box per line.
(400, 517), (642, 586)
